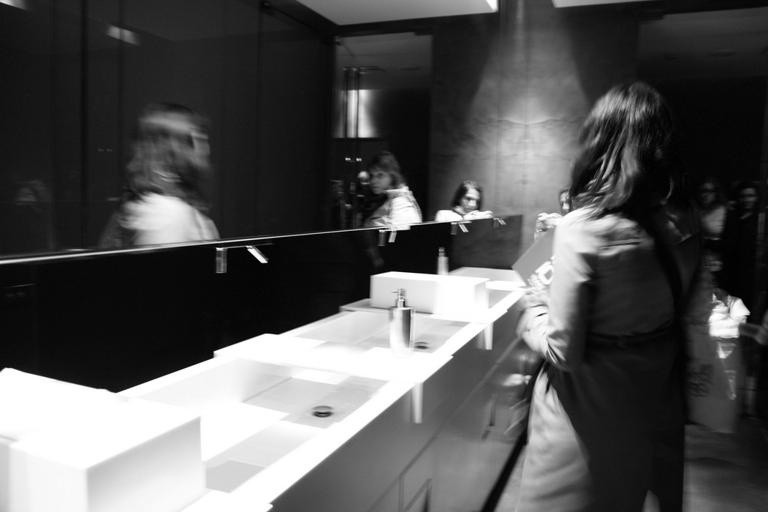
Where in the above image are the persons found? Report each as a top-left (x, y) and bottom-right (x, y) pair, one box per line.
(361, 150), (421, 230)
(434, 180), (493, 222)
(513, 80), (685, 512)
(96, 102), (224, 251)
(687, 172), (726, 250)
(721, 182), (767, 304)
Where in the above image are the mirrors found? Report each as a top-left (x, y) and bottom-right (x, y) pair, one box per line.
(1, 2), (501, 254)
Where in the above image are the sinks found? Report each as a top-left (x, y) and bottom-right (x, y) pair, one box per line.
(106, 332), (450, 512)
(338, 274), (523, 348)
(292, 309), (486, 421)
